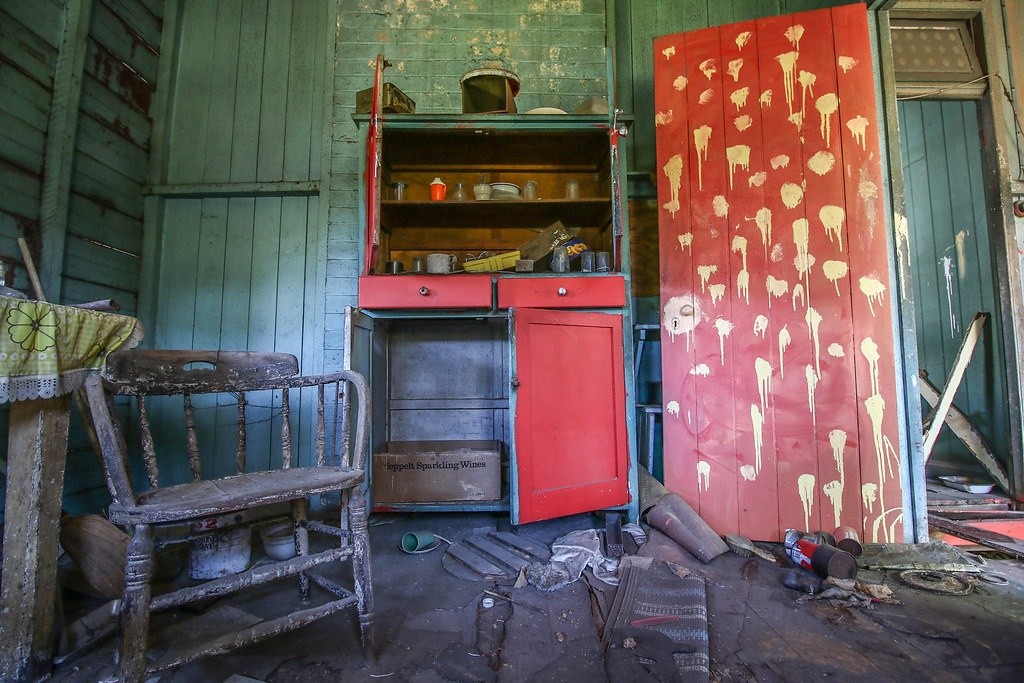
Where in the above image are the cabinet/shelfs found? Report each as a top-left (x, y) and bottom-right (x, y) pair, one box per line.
(340, 112), (644, 561)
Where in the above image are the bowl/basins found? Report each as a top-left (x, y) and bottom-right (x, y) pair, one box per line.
(260, 523), (295, 561)
(490, 183), (522, 195)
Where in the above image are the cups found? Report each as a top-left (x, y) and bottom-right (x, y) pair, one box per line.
(385, 262), (403, 274)
(473, 184), (493, 200)
(581, 252), (595, 273)
(402, 531), (434, 554)
(390, 182), (408, 200)
(413, 258), (425, 273)
(427, 254), (456, 274)
(566, 181), (579, 199)
(596, 252), (610, 272)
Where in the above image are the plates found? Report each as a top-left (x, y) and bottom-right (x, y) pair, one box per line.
(398, 538), (441, 554)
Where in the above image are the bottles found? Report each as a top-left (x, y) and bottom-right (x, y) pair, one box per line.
(553, 246), (570, 273)
(524, 180), (536, 200)
(453, 183), (468, 200)
(430, 178), (446, 201)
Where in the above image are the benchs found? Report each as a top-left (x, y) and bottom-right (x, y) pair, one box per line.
(90, 351), (374, 683)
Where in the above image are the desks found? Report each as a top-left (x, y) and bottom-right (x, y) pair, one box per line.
(1, 293), (143, 682)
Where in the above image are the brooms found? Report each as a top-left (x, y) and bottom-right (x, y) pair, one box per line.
(59, 510), (133, 601)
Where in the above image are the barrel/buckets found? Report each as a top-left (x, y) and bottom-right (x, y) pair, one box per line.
(784, 526), (863, 580)
(188, 508), (254, 579)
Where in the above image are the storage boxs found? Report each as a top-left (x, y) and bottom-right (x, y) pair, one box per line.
(356, 82), (416, 115)
(371, 440), (503, 503)
